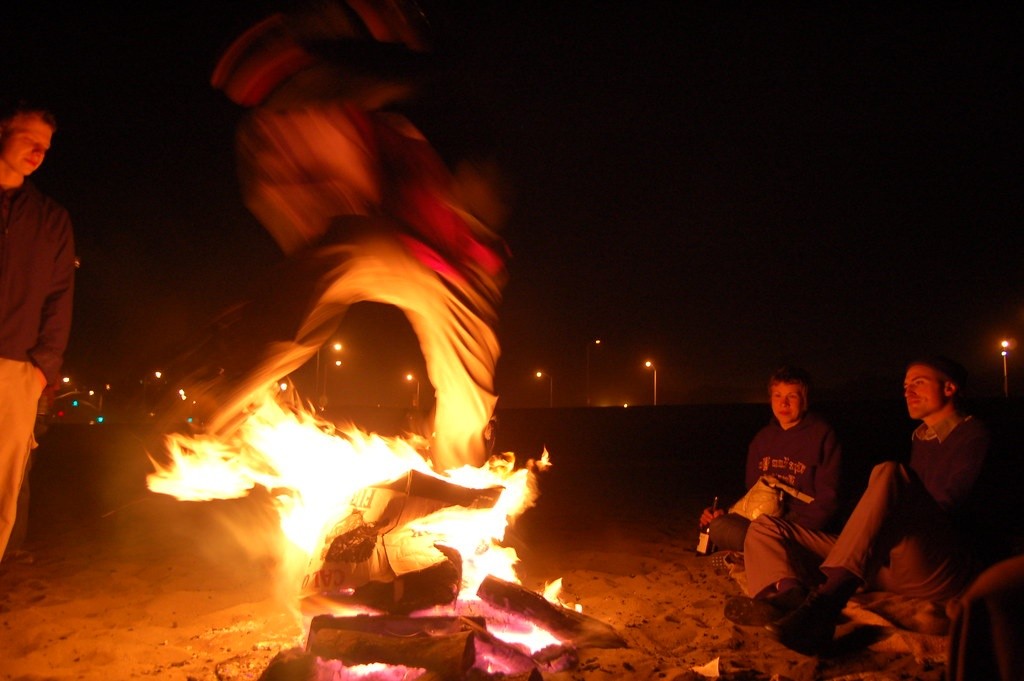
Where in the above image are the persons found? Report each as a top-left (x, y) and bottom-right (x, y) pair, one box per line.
(697, 366), (846, 552)
(724, 351), (1024, 679)
(0, 108), (76, 566)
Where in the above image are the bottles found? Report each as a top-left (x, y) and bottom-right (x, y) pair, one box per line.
(693, 496), (720, 557)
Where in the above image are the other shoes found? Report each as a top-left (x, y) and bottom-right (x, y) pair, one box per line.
(719, 576), (857, 646)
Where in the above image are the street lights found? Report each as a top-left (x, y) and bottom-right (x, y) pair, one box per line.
(536, 369), (554, 407)
(316, 341), (343, 408)
(585, 338), (601, 409)
(406, 374), (420, 412)
(1001, 340), (1009, 398)
(644, 360), (657, 405)
